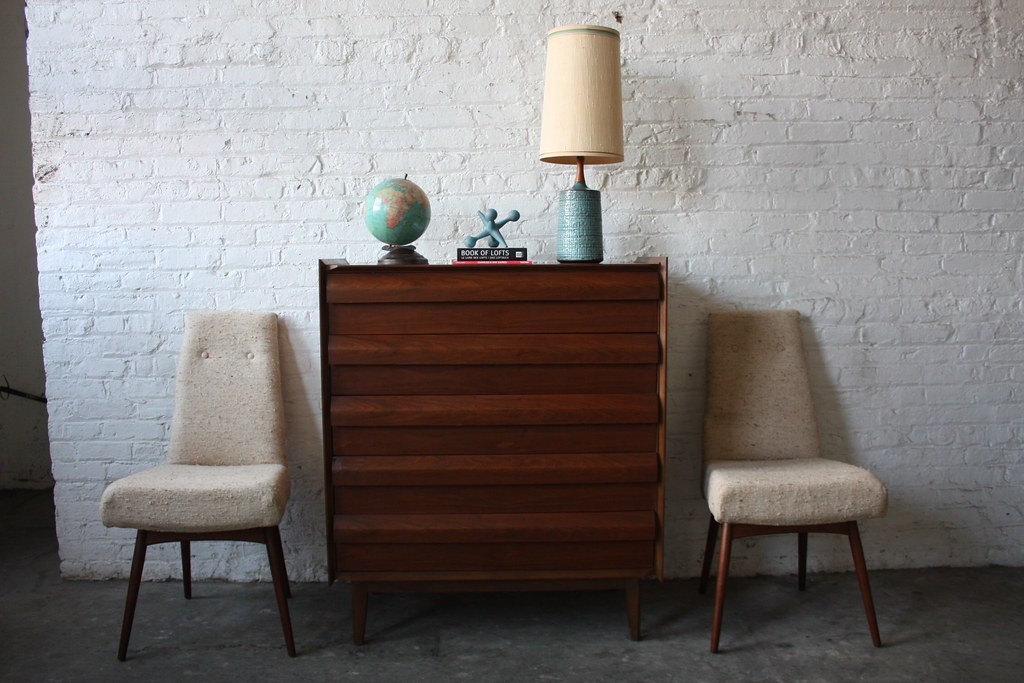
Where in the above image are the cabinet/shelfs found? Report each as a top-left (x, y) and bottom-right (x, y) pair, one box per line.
(317, 256), (669, 647)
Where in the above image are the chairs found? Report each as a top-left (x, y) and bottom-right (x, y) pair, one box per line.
(101, 312), (299, 661)
(698, 310), (888, 654)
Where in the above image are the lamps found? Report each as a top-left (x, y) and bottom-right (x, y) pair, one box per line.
(539, 24), (624, 265)
